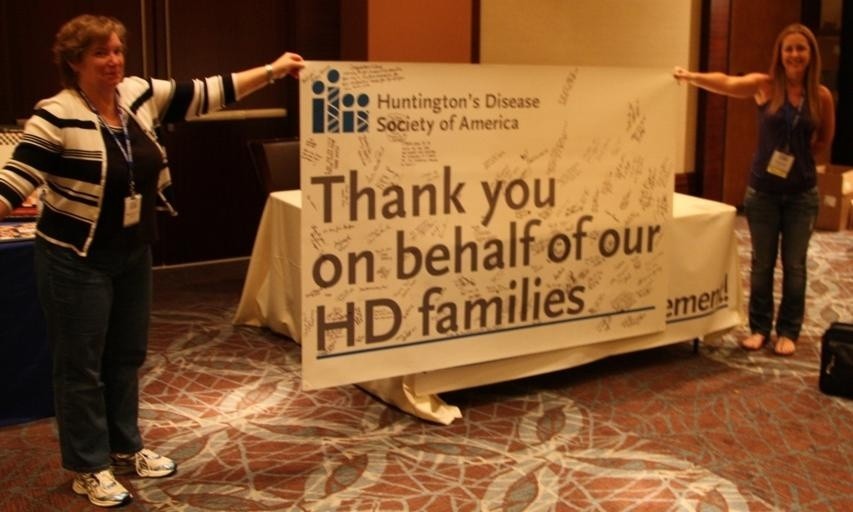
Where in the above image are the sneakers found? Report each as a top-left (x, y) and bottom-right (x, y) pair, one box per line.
(109, 447), (176, 479)
(774, 335), (796, 355)
(70, 466), (134, 508)
(740, 331), (766, 351)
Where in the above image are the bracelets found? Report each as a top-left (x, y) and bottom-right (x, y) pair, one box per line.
(265, 63), (277, 88)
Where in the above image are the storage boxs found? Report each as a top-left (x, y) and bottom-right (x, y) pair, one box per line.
(817, 163), (853, 233)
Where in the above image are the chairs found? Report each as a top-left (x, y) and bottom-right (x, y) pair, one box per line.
(251, 135), (300, 193)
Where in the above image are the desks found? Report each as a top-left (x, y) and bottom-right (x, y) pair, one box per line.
(229, 187), (747, 427)
(2, 241), (58, 425)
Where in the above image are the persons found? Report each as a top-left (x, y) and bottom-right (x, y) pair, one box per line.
(0, 11), (305, 509)
(672, 21), (836, 357)
